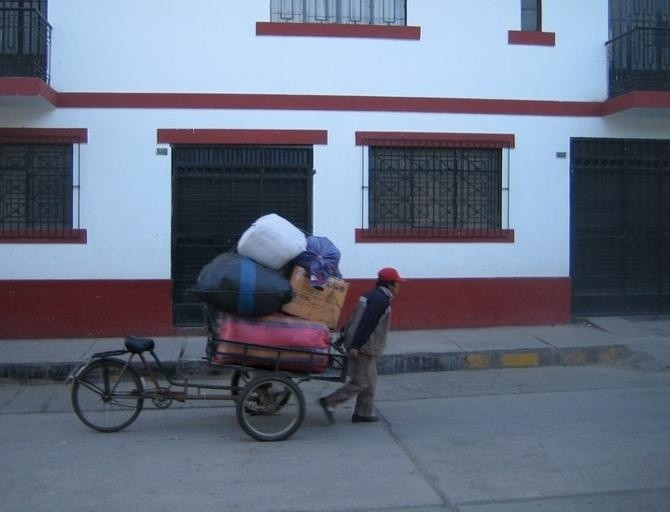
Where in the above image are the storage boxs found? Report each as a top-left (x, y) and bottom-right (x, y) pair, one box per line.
(281, 264), (350, 331)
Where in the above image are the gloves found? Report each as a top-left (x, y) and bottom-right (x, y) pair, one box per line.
(349, 347), (358, 356)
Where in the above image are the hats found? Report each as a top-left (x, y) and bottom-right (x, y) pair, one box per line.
(377, 268), (407, 285)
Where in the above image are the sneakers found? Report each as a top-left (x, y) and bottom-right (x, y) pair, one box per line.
(351, 413), (380, 424)
(319, 396), (336, 422)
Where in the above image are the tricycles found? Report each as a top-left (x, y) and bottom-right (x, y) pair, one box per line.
(63, 318), (356, 442)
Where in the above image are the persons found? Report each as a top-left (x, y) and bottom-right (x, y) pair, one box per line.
(313, 268), (406, 423)
